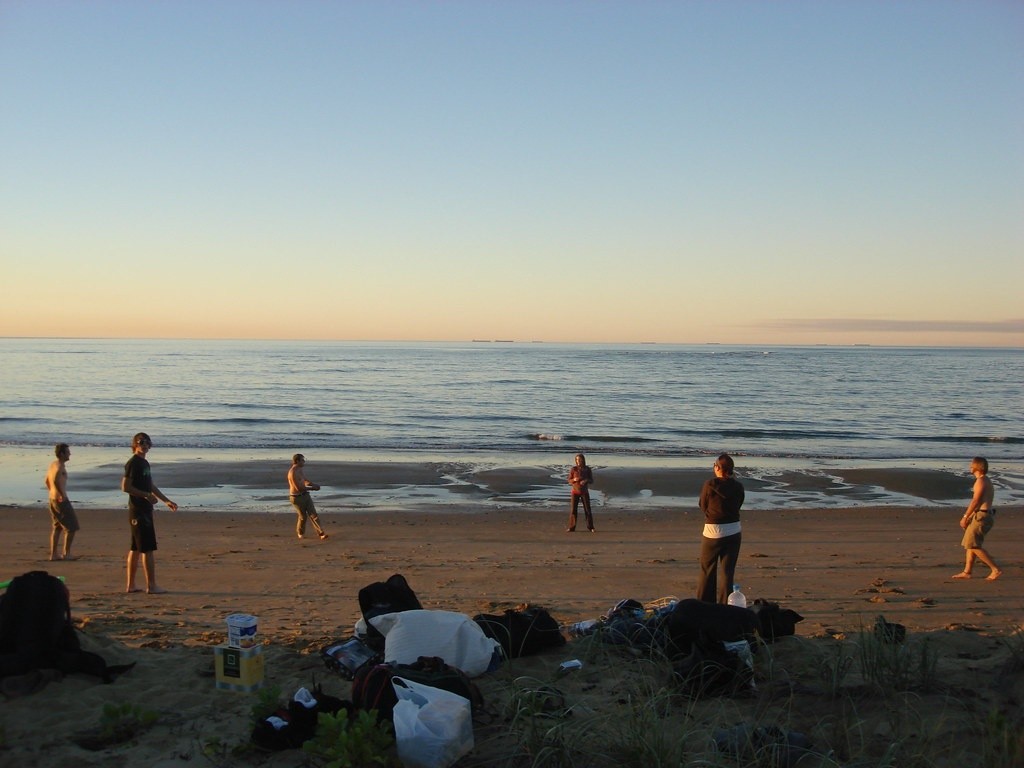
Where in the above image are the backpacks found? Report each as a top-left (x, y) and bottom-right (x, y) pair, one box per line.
(0, 570), (72, 678)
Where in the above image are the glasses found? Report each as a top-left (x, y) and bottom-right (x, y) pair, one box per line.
(136, 441), (150, 445)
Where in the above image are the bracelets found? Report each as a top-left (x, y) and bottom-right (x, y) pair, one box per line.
(963, 514), (967, 518)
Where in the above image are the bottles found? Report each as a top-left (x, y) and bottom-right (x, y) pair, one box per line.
(727, 585), (746, 608)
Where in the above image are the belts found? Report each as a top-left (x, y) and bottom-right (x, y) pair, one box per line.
(290, 492), (310, 497)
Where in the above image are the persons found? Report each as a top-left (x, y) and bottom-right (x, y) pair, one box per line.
(565, 453), (597, 532)
(44, 442), (84, 561)
(952, 456), (1002, 580)
(696, 452), (746, 604)
(120, 431), (179, 595)
(287, 453), (332, 540)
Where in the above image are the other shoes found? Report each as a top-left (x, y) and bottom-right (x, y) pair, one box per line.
(298, 533), (303, 539)
(321, 534), (328, 540)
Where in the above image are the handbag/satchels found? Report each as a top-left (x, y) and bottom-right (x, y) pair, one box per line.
(358, 574), (424, 650)
(472, 605), (566, 656)
(351, 656), (485, 733)
(390, 675), (474, 768)
